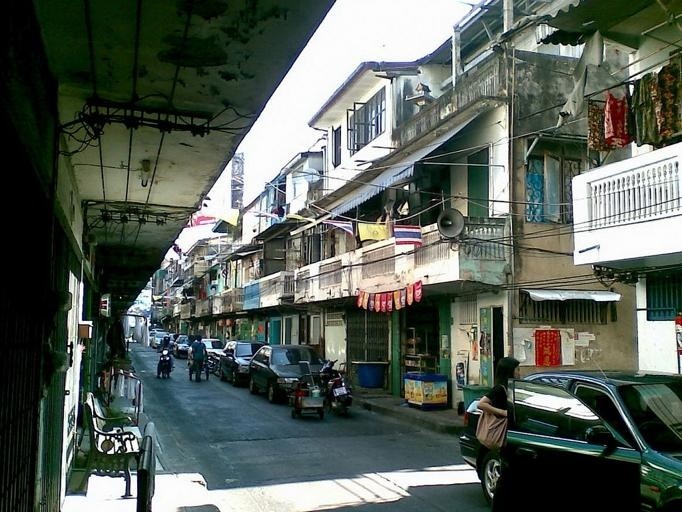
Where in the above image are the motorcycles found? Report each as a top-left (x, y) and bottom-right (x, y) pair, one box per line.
(290, 371), (326, 419)
(204, 351), (220, 377)
(157, 346), (175, 379)
(318, 359), (353, 415)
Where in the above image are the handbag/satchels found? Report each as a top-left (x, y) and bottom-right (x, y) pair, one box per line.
(475, 408), (507, 450)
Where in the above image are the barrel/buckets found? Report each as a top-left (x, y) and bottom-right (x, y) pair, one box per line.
(350, 360), (391, 388)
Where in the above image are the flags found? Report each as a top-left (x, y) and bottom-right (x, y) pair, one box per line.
(202, 206), (423, 245)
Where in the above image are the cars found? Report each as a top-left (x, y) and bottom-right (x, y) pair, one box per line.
(150, 328), (190, 357)
(248, 342), (329, 405)
(187, 338), (227, 367)
(457, 368), (682, 509)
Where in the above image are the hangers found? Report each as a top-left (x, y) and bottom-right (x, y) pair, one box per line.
(666, 57), (674, 67)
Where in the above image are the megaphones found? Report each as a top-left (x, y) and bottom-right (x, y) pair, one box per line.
(437, 208), (465, 238)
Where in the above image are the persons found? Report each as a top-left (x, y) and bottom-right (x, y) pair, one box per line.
(157, 334), (208, 383)
(477, 357), (520, 512)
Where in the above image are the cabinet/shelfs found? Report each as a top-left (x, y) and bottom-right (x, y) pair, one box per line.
(405, 326), (429, 357)
(402, 355), (438, 395)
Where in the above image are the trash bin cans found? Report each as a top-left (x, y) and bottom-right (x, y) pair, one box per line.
(462, 384), (492, 414)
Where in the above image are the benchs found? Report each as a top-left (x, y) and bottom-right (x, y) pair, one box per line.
(76, 389), (142, 497)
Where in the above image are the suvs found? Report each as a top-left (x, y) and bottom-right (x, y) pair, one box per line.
(218, 340), (272, 387)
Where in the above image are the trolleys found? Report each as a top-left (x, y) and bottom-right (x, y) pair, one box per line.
(186, 353), (210, 381)
